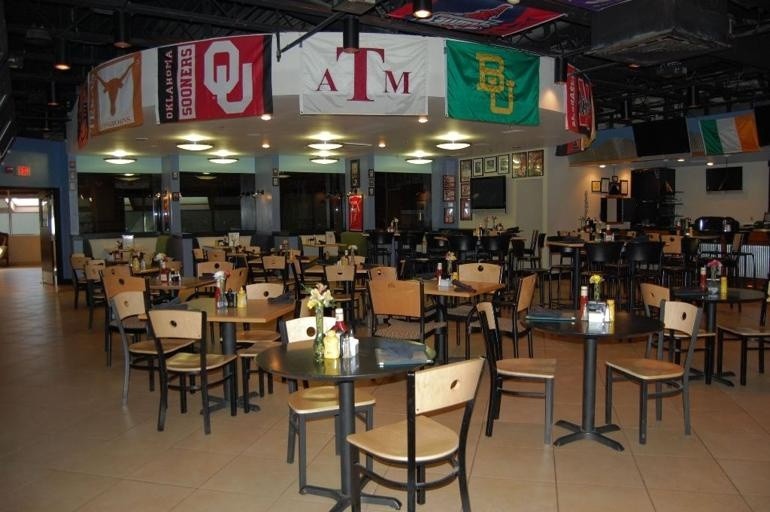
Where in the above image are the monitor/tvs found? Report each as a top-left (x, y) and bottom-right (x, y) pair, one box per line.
(706, 166), (743, 191)
(631, 117), (691, 156)
(470, 175), (507, 210)
(630, 168), (676, 199)
(754, 104), (770, 148)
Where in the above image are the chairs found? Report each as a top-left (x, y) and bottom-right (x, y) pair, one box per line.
(0, 232), (9, 267)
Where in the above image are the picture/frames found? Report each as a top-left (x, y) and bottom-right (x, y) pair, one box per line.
(592, 175), (629, 196)
(368, 168), (375, 196)
(350, 159), (360, 189)
(273, 177), (279, 186)
(348, 194), (363, 233)
(442, 149), (544, 223)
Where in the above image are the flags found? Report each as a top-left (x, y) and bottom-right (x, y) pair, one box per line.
(698, 114), (760, 156)
(76, 86), (89, 147)
(556, 92), (597, 156)
(440, 39), (540, 126)
(88, 52), (142, 137)
(156, 35), (274, 123)
(565, 65), (591, 139)
(299, 32), (428, 115)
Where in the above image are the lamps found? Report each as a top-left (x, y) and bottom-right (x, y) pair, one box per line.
(621, 99), (631, 121)
(554, 38), (566, 83)
(412, 0), (431, 18)
(726, 12), (737, 41)
(688, 85), (702, 109)
(342, 14), (360, 53)
(7, 8), (133, 134)
(9, 197), (48, 212)
(252, 190), (265, 199)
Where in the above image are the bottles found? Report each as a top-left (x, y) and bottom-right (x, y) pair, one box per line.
(698, 265), (728, 295)
(436, 261), (458, 288)
(579, 285), (589, 321)
(474, 223), (483, 236)
(603, 298), (616, 322)
(221, 233), (350, 266)
(325, 357), (359, 375)
(325, 306), (360, 359)
(675, 215), (693, 239)
(585, 216), (614, 242)
(132, 251), (248, 308)
